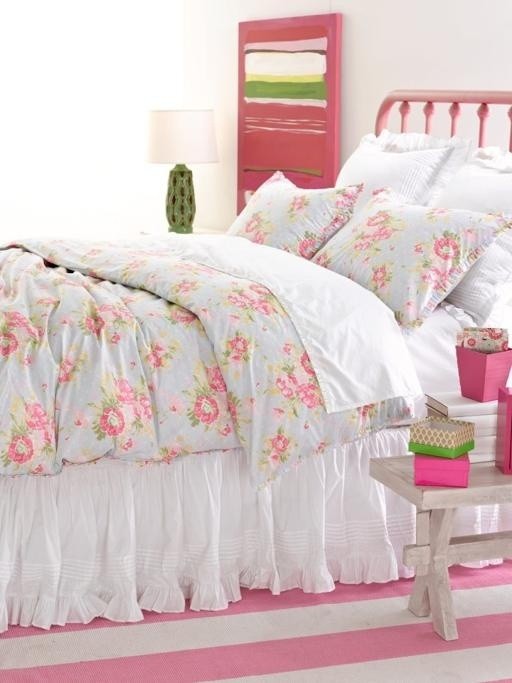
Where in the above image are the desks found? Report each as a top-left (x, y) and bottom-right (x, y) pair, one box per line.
(370, 458), (512, 641)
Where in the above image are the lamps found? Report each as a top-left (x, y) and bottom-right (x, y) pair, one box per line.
(144, 109), (221, 232)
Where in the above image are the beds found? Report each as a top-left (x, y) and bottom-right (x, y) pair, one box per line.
(0, 89), (512, 636)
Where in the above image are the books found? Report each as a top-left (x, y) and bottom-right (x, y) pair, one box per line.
(423, 391), (499, 465)
